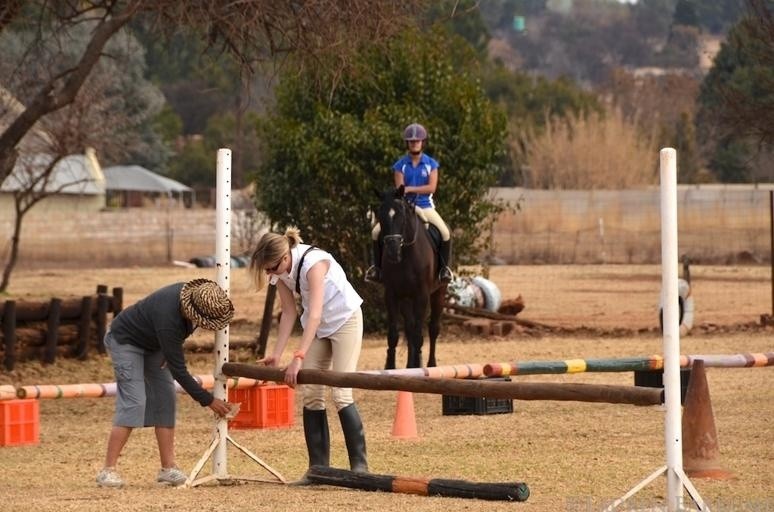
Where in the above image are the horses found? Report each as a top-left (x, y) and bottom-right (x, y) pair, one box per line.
(366, 183), (452, 371)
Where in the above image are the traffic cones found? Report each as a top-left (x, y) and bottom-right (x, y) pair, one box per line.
(390, 390), (418, 440)
(664, 358), (737, 481)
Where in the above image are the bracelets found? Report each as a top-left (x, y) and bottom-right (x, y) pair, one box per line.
(293, 350), (304, 360)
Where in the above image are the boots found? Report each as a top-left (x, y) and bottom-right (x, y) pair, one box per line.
(338, 402), (368, 473)
(439, 237), (452, 281)
(367, 239), (380, 281)
(287, 406), (330, 486)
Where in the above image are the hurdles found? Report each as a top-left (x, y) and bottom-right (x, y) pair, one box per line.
(183, 147), (712, 512)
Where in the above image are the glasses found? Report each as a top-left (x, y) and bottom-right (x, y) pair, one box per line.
(265, 253), (286, 271)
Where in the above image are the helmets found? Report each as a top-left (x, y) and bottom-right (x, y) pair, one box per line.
(404, 123), (427, 141)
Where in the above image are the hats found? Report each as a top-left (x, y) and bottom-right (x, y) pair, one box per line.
(179, 277), (235, 330)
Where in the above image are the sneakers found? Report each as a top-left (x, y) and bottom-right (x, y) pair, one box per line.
(157, 465), (187, 486)
(96, 470), (125, 487)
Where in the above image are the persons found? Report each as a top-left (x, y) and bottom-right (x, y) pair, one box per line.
(366, 123), (452, 281)
(95, 277), (235, 488)
(249, 225), (369, 487)
